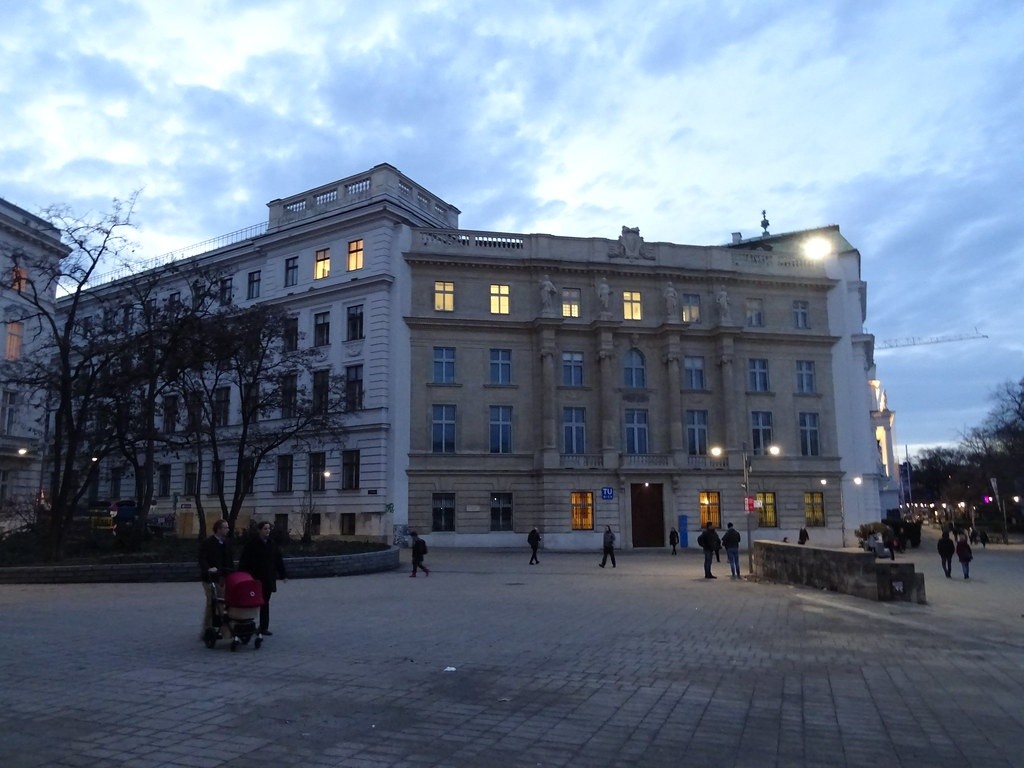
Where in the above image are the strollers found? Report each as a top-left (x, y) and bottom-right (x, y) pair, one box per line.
(203, 566), (263, 653)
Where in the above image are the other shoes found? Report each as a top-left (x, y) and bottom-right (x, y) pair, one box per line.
(409, 573), (417, 577)
(424, 569), (430, 576)
(258, 629), (273, 635)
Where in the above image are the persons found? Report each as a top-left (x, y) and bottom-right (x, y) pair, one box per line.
(798, 524), (810, 545)
(197, 519), (234, 640)
(865, 511), (990, 561)
(527, 526), (542, 565)
(598, 524), (616, 568)
(783, 537), (789, 543)
(240, 522), (285, 636)
(712, 528), (722, 562)
(937, 531), (955, 578)
(696, 521), (721, 579)
(722, 522), (743, 580)
(409, 530), (431, 577)
(956, 533), (974, 580)
(669, 527), (680, 555)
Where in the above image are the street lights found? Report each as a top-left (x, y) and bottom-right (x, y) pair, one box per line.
(819, 477), (863, 547)
(309, 461), (330, 537)
(898, 495), (1020, 544)
(18, 446), (47, 525)
(711, 442), (782, 572)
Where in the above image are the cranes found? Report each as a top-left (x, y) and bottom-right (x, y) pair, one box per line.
(862, 327), (989, 349)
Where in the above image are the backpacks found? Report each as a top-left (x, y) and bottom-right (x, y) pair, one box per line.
(419, 539), (427, 554)
